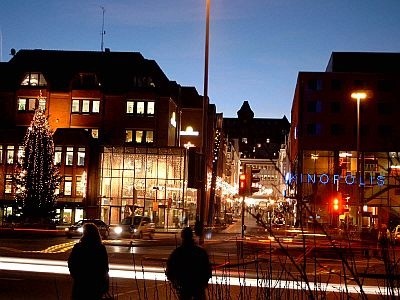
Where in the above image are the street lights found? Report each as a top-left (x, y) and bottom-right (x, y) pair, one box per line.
(350, 90), (368, 231)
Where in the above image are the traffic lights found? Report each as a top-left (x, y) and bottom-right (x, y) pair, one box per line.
(332, 197), (340, 212)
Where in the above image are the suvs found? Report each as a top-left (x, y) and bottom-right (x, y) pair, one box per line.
(113, 215), (156, 239)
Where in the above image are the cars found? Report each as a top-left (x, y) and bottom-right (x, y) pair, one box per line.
(65, 218), (111, 239)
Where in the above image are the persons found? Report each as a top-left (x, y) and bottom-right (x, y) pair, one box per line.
(165, 228), (212, 300)
(68, 223), (109, 300)
(194, 217), (204, 244)
(360, 225), (392, 259)
